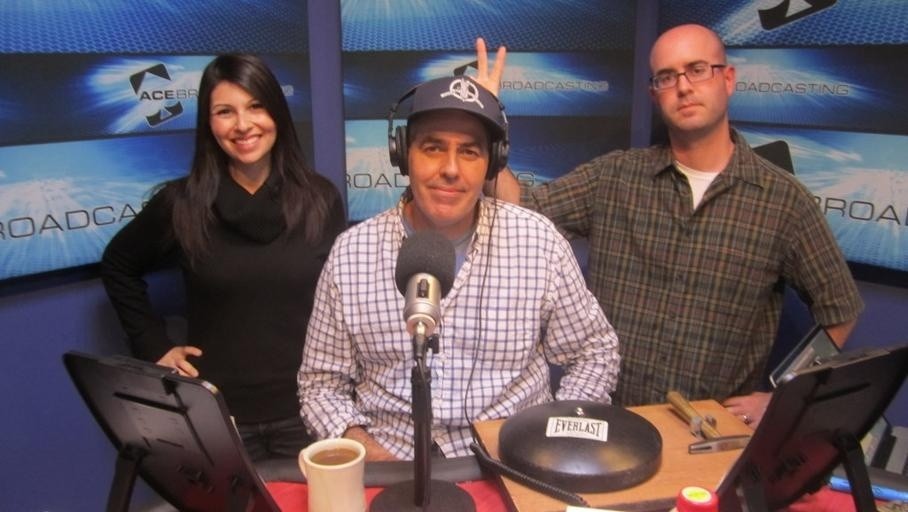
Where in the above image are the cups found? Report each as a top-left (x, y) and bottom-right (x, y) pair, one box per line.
(299, 438), (367, 512)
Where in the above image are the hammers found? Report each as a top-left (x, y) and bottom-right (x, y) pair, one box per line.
(666, 390), (751, 454)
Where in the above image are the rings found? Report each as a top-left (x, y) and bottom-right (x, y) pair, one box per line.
(740, 412), (752, 426)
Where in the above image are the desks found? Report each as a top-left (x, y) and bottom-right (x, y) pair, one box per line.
(150, 456), (908, 512)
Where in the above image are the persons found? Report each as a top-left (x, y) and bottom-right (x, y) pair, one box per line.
(296, 73), (623, 463)
(469, 19), (868, 435)
(99, 50), (348, 466)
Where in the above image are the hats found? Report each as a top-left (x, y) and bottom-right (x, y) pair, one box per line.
(403, 74), (507, 143)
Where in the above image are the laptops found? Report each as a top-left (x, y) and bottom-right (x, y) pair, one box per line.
(769, 323), (908, 494)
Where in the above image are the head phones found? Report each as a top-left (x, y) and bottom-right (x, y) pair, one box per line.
(388, 83), (510, 180)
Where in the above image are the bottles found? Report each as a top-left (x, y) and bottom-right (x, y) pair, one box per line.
(669, 486), (720, 512)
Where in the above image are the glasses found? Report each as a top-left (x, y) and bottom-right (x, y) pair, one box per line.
(648, 64), (726, 90)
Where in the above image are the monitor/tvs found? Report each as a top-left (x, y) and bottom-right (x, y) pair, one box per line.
(714, 342), (908, 512)
(63, 350), (282, 512)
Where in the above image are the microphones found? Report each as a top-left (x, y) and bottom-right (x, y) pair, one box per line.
(395, 231), (456, 337)
